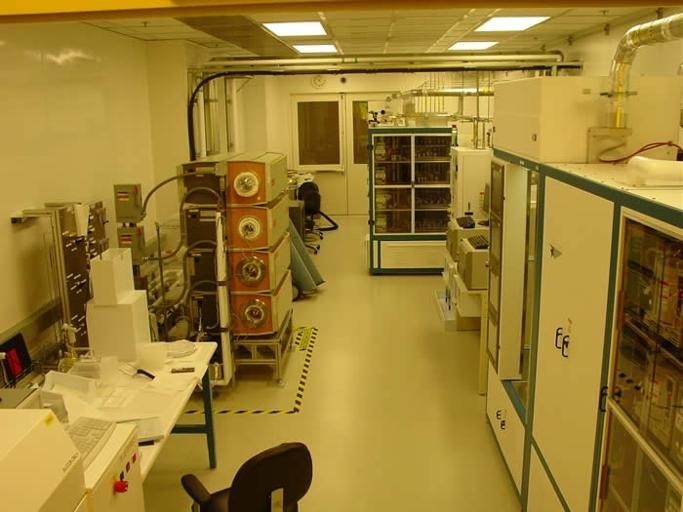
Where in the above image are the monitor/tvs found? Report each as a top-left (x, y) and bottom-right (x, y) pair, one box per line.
(478, 183), (490, 226)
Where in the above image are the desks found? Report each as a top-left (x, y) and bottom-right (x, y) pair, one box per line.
(56, 338), (219, 486)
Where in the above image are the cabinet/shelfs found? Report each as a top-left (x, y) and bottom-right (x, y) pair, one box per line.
(483, 145), (683, 512)
(365, 125), (451, 275)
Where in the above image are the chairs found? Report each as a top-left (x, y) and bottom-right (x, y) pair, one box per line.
(298, 181), (323, 243)
(180, 438), (315, 512)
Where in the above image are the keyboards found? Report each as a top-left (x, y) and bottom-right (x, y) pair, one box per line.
(467, 235), (489, 249)
(61, 416), (117, 472)
(456, 217), (475, 228)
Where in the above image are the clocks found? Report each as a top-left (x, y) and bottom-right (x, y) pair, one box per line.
(311, 73), (329, 89)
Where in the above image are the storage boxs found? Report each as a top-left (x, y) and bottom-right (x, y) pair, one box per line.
(89, 247), (134, 308)
(84, 289), (152, 362)
